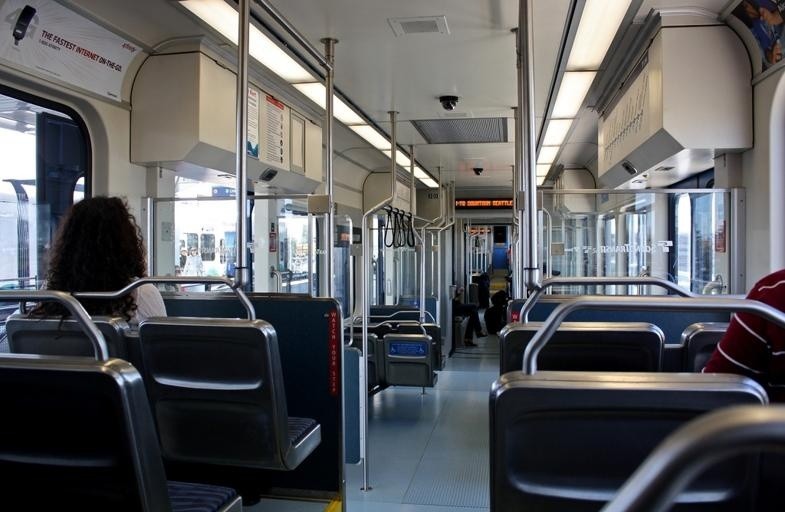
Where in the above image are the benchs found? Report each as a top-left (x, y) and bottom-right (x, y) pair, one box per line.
(2, 277), (324, 505)
(489, 277), (785, 512)
(3, 288), (243, 509)
(343, 310), (446, 394)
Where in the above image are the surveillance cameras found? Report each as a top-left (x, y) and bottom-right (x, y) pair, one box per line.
(473, 168), (484, 176)
(440, 96), (457, 113)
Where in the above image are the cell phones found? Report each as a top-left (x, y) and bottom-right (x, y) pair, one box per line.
(12, 5), (38, 39)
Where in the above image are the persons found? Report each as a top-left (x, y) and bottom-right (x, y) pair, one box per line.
(178, 246), (188, 273)
(183, 246), (204, 276)
(451, 281), (488, 349)
(701, 268), (785, 409)
(8, 194), (167, 329)
